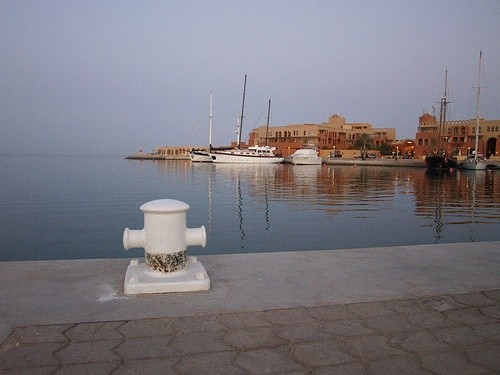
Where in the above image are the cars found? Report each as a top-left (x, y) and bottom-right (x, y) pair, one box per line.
(326, 148), (485, 163)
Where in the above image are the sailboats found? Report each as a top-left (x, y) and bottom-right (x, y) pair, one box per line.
(423, 69), (462, 168)
(187, 93), (250, 162)
(459, 47), (489, 171)
(207, 73), (286, 165)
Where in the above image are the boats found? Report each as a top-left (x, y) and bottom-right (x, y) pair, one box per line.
(291, 148), (323, 165)
